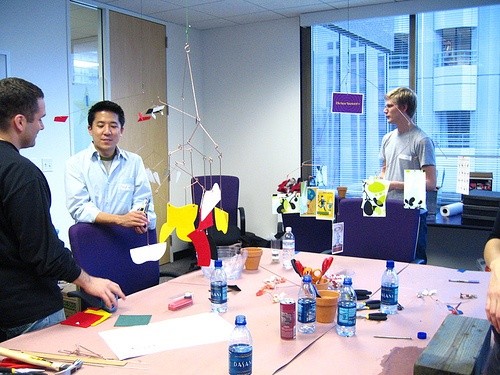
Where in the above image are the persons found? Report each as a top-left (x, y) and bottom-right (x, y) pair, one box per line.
(484, 227), (500, 333)
(65, 100), (156, 233)
(378, 87), (437, 265)
(0, 77), (127, 342)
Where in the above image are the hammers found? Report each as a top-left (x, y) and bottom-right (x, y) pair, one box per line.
(0, 347), (83, 375)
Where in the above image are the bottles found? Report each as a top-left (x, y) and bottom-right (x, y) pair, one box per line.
(336, 276), (357, 338)
(382, 261), (399, 315)
(297, 276), (317, 334)
(210, 260), (227, 312)
(228, 314), (252, 375)
(282, 227), (296, 269)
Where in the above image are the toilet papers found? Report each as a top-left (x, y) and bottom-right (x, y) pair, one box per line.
(439, 202), (464, 217)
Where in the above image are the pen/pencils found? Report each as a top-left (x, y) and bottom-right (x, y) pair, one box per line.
(374, 336), (411, 340)
(449, 279), (479, 283)
(291, 259), (302, 278)
(295, 259), (305, 277)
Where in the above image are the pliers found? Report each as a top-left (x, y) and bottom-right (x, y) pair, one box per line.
(355, 290), (372, 300)
(358, 300), (381, 311)
(356, 312), (387, 320)
(0, 367), (48, 375)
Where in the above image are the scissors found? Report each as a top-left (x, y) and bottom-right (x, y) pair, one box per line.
(302, 267), (322, 284)
(317, 257), (334, 284)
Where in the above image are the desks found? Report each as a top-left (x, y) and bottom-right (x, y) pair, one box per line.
(0, 247), (500, 375)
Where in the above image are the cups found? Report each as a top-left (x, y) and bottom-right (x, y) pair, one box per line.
(337, 186), (347, 198)
(270, 239), (280, 263)
(317, 279), (329, 289)
(240, 248), (263, 270)
(216, 245), (239, 259)
(317, 290), (339, 323)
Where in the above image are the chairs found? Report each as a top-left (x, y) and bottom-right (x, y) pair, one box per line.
(320, 198), (427, 266)
(69, 221), (160, 311)
(189, 175), (246, 269)
